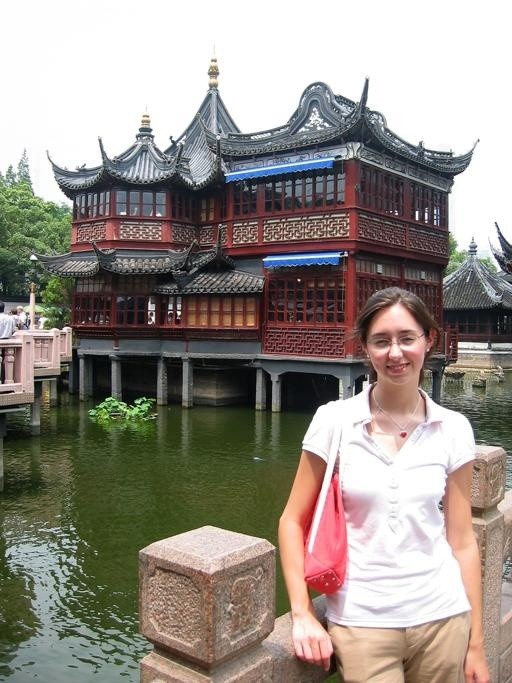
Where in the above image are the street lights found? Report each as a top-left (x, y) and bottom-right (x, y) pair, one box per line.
(28, 281), (38, 329)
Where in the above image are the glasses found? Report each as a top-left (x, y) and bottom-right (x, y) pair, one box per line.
(367, 332), (425, 348)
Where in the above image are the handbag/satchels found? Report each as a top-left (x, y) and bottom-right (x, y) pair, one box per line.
(304, 471), (348, 596)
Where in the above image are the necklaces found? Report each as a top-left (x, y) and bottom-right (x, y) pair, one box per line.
(371, 380), (424, 440)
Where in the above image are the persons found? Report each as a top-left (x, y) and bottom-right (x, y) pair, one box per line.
(0, 302), (32, 338)
(279, 286), (492, 683)
(149, 306), (184, 326)
(38, 314), (51, 329)
